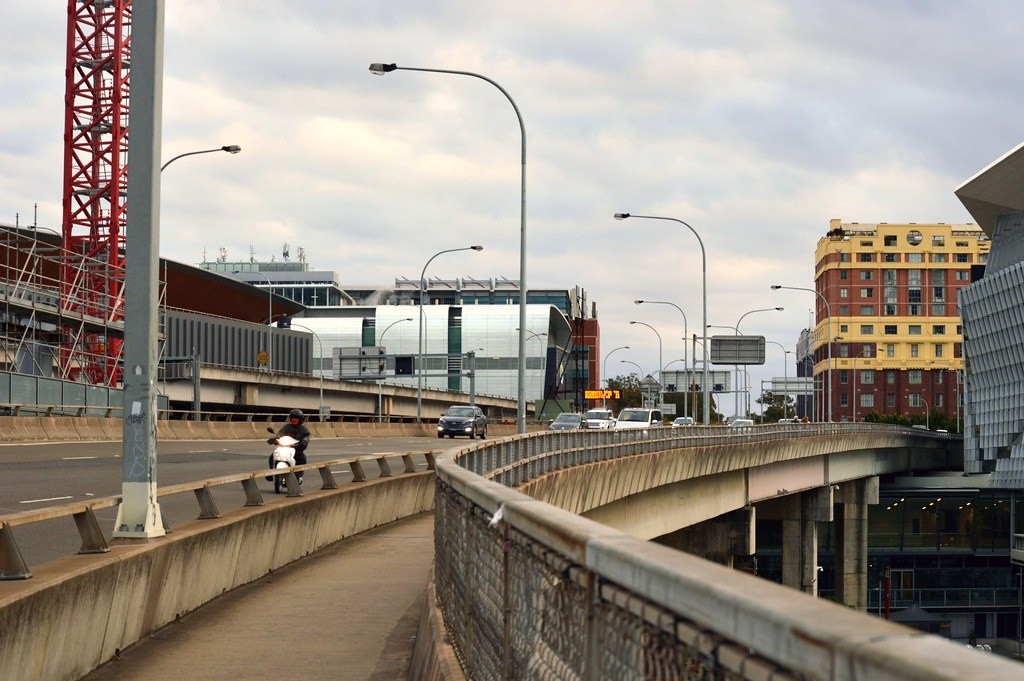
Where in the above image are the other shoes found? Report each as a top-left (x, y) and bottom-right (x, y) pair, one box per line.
(265, 476), (273, 481)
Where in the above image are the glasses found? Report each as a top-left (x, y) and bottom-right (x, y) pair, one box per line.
(291, 415), (299, 419)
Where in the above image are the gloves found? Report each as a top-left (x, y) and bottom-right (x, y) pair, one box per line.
(268, 438), (278, 444)
(299, 441), (308, 449)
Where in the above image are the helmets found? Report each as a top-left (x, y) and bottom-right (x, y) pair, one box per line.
(288, 409), (304, 425)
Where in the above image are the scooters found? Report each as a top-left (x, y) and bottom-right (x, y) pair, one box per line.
(266, 427), (311, 495)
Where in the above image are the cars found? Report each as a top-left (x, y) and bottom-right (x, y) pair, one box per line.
(726, 418), (756, 435)
(584, 409), (617, 431)
(777, 419), (810, 424)
(548, 411), (585, 431)
(669, 416), (695, 435)
(613, 407), (664, 441)
(437, 404), (489, 439)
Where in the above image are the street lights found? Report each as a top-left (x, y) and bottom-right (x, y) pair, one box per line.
(734, 307), (784, 420)
(804, 336), (845, 424)
(233, 270), (273, 375)
(911, 397), (929, 430)
(367, 62), (529, 437)
(930, 361), (959, 434)
(771, 285), (833, 423)
(629, 321), (662, 409)
(515, 327), (544, 400)
(706, 325), (747, 419)
(603, 346), (630, 410)
(621, 360), (644, 380)
(286, 322), (324, 422)
(377, 317), (414, 423)
(852, 347), (885, 423)
(634, 300), (688, 426)
(415, 245), (484, 424)
(613, 213), (709, 428)
(786, 350), (815, 423)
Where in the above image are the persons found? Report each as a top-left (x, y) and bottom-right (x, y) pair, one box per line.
(801, 416), (808, 423)
(265, 409), (310, 481)
(791, 415), (799, 430)
